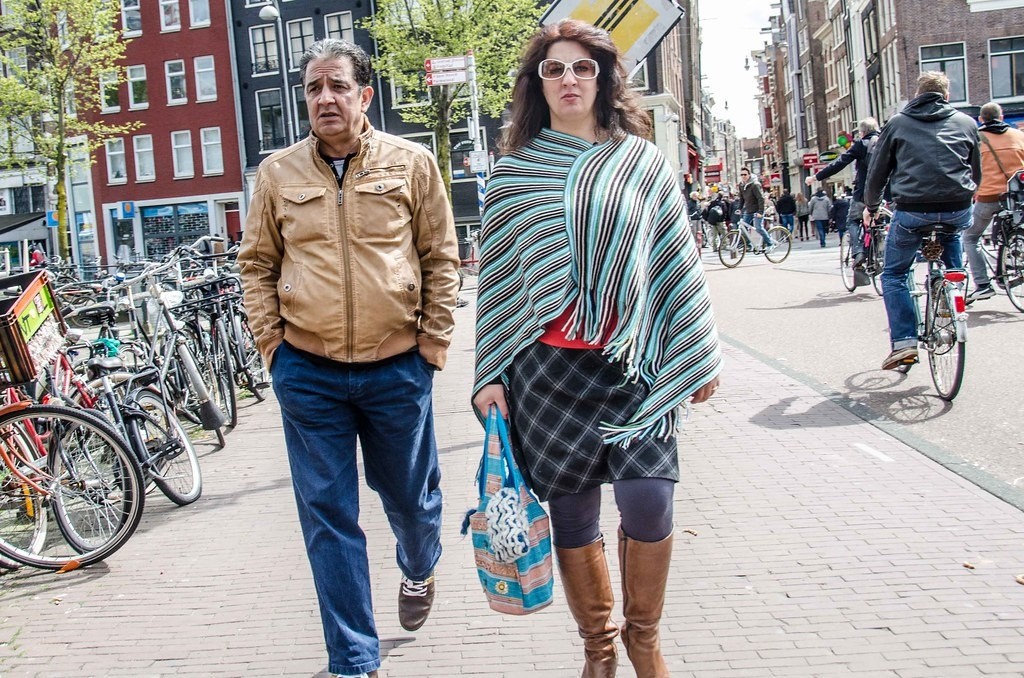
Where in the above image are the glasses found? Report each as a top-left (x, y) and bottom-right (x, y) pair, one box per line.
(538, 59), (600, 80)
(741, 174), (746, 176)
(859, 131), (862, 134)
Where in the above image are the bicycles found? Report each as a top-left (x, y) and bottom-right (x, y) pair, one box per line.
(957, 169), (1024, 313)
(0, 235), (272, 571)
(866, 206), (970, 401)
(718, 214), (792, 268)
(840, 197), (891, 297)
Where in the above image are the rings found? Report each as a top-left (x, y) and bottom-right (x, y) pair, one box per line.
(711, 386), (718, 391)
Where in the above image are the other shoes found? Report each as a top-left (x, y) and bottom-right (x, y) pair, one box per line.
(765, 240), (777, 253)
(713, 248), (717, 251)
(739, 245), (751, 252)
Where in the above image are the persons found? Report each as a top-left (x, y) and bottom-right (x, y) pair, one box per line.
(471, 19), (722, 678)
(683, 167), (854, 258)
(239, 39), (462, 678)
(805, 117), (893, 269)
(117, 241), (131, 261)
(863, 71), (981, 370)
(962, 102), (1024, 298)
(31, 246), (45, 266)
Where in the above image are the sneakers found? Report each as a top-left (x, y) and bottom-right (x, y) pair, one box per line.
(966, 284), (995, 299)
(882, 348), (918, 369)
(939, 301), (949, 313)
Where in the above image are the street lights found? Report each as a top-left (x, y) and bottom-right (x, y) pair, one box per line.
(259, 0), (296, 146)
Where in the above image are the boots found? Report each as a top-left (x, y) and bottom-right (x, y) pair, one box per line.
(618, 522), (675, 678)
(553, 533), (620, 678)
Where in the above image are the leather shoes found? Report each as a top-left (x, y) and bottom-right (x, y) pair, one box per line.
(398, 571), (435, 631)
(331, 670), (378, 678)
(852, 253), (865, 270)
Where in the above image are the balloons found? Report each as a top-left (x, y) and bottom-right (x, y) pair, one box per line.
(838, 130), (852, 150)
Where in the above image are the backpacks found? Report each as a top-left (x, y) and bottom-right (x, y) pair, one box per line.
(862, 135), (879, 167)
(708, 201), (724, 225)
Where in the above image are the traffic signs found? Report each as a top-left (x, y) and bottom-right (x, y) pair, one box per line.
(424, 56), (468, 86)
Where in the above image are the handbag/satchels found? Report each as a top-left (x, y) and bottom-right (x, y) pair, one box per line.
(470, 402), (554, 615)
